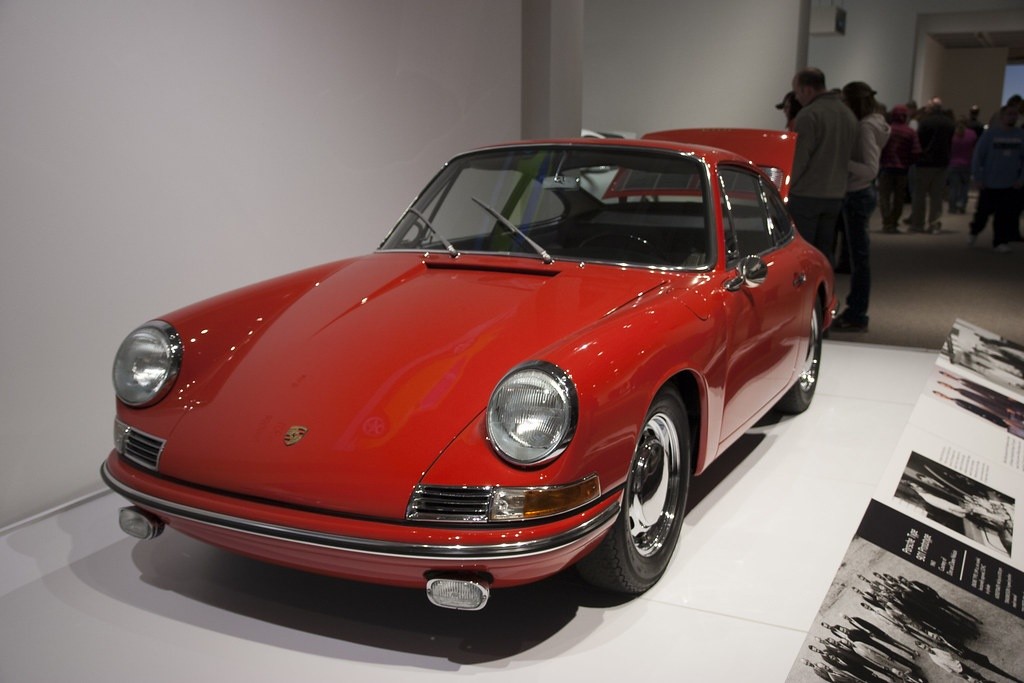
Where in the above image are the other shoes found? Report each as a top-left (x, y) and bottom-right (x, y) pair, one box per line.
(910, 226), (925, 233)
(831, 315), (869, 333)
(929, 224), (940, 234)
(959, 207), (965, 213)
(947, 207), (957, 213)
(993, 243), (1011, 253)
(835, 263), (851, 275)
(967, 234), (977, 244)
(903, 218), (913, 224)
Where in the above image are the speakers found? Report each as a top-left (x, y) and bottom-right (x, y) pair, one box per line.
(810, 6), (847, 36)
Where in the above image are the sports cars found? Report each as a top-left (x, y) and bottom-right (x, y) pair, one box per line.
(99, 127), (840, 614)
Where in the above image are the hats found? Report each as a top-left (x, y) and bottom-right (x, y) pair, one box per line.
(774, 92), (803, 109)
(892, 105), (908, 115)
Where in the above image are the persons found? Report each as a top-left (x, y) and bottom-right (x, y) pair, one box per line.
(992, 95), (1024, 242)
(908, 481), (992, 528)
(789, 67), (863, 338)
(915, 473), (979, 499)
(775, 92), (803, 129)
(909, 97), (954, 233)
(801, 572), (1024, 683)
(968, 105), (1021, 253)
(829, 81), (892, 332)
(947, 115), (977, 214)
(965, 105), (983, 138)
(907, 101), (917, 113)
(878, 106), (922, 233)
(933, 332), (1024, 430)
